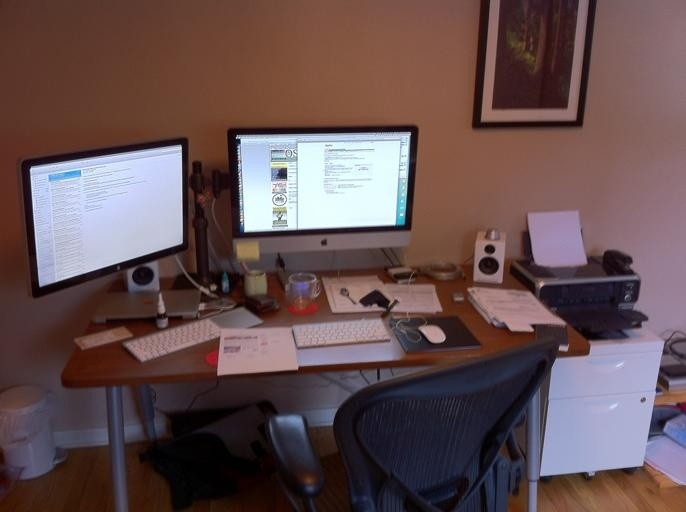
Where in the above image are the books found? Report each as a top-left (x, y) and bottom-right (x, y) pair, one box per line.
(321, 274), (443, 315)
(643, 350), (686, 490)
(216, 326), (300, 378)
(465, 284), (568, 334)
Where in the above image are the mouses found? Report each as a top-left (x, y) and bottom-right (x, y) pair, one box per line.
(417, 324), (448, 345)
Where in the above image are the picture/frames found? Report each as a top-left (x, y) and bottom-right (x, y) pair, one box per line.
(472, 1), (597, 130)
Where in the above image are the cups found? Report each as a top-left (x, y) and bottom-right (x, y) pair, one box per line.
(285, 273), (321, 315)
(242, 270), (268, 299)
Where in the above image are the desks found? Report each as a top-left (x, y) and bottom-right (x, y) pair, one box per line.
(60, 265), (589, 510)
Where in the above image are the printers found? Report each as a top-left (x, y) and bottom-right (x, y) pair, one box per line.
(510, 228), (649, 341)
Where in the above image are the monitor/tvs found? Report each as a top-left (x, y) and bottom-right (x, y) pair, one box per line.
(18, 135), (194, 301)
(226, 123), (419, 255)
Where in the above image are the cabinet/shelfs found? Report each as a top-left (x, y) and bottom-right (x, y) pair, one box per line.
(538, 328), (663, 484)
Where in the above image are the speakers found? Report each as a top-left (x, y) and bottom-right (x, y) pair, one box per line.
(472, 228), (507, 285)
(123, 259), (161, 293)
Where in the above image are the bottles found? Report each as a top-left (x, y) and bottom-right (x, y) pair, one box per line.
(157, 292), (168, 329)
(221, 272), (230, 292)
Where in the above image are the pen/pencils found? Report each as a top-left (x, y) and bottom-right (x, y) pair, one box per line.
(241, 261), (250, 276)
(381, 300), (398, 318)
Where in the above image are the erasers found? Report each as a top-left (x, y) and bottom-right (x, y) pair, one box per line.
(453, 292), (464, 303)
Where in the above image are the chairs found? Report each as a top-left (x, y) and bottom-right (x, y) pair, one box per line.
(249, 339), (562, 512)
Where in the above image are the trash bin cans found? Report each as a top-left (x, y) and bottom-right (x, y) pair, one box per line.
(0, 385), (68, 480)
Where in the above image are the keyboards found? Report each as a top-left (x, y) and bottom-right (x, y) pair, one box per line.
(121, 317), (222, 364)
(292, 318), (391, 349)
